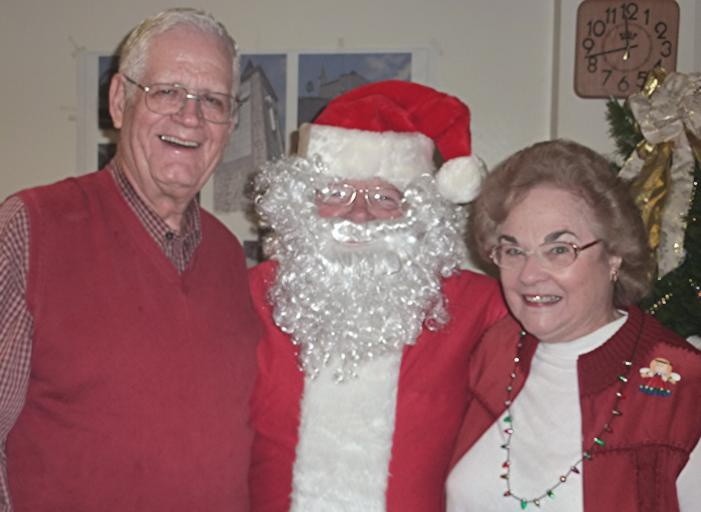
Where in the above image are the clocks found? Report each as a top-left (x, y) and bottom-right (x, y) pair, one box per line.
(574, 0), (680, 99)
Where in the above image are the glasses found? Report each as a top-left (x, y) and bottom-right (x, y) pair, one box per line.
(488, 237), (605, 269)
(313, 182), (411, 212)
(119, 71), (241, 128)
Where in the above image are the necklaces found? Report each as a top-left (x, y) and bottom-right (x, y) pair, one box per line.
(504, 312), (650, 505)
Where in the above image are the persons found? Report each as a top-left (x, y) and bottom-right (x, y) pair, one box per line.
(1, 5), (260, 512)
(242, 78), (516, 512)
(433, 137), (701, 511)
